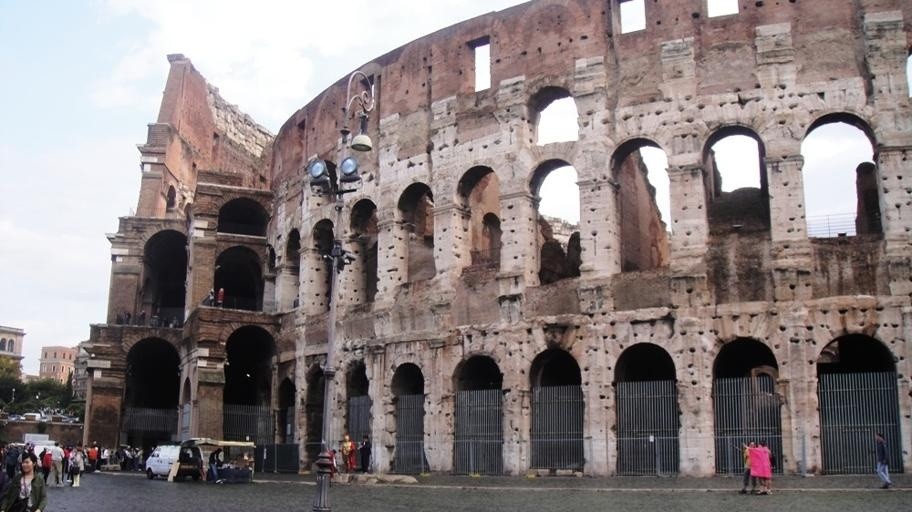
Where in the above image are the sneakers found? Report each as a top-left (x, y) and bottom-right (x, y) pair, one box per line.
(880, 482), (892, 489)
(216, 479), (223, 484)
(738, 489), (774, 495)
(45, 479), (80, 488)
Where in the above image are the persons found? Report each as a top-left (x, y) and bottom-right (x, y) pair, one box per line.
(871, 432), (893, 490)
(328, 447), (337, 488)
(152, 311), (161, 327)
(137, 309), (145, 325)
(171, 315), (179, 327)
(208, 447), (226, 484)
(742, 440), (774, 495)
(207, 287), (215, 307)
(123, 310), (131, 325)
(359, 434), (371, 475)
(1, 440), (155, 489)
(1, 453), (49, 512)
(196, 455), (205, 481)
(217, 286), (224, 308)
(737, 441), (758, 495)
(31, 404), (75, 417)
(339, 432), (358, 472)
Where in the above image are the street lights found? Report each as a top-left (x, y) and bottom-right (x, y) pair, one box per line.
(305, 70), (378, 511)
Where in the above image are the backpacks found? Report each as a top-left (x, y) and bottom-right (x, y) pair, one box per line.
(769, 449), (777, 467)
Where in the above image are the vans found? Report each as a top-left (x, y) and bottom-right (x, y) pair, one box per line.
(144, 443), (204, 480)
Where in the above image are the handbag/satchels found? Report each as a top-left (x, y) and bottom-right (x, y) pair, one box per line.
(4, 496), (29, 512)
(70, 464), (80, 475)
